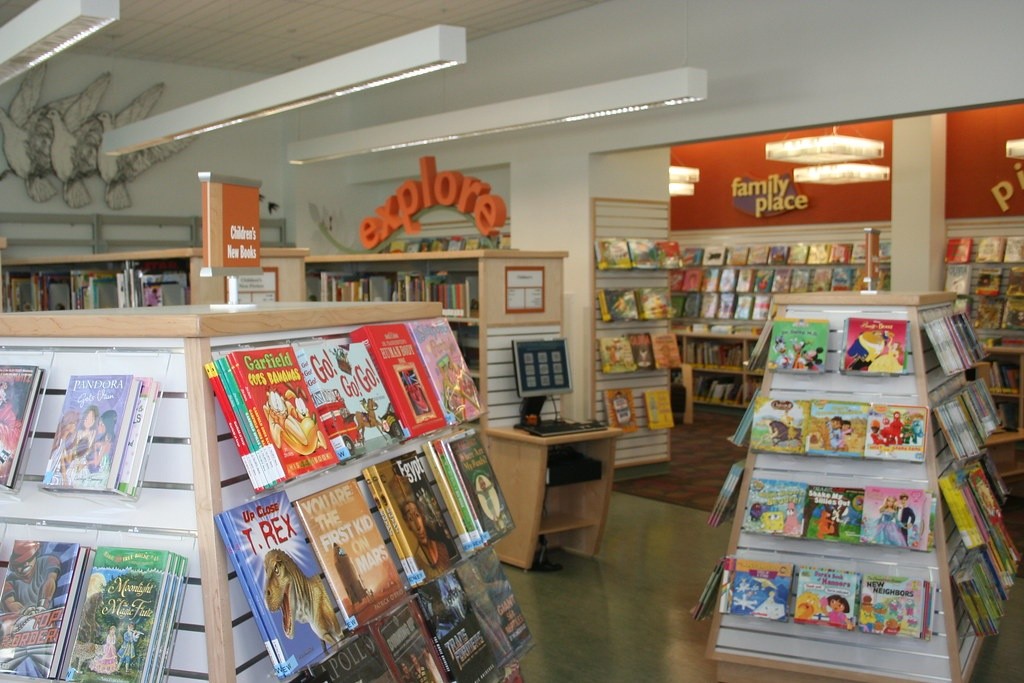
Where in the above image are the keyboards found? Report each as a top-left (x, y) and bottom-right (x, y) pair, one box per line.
(529, 423), (606, 436)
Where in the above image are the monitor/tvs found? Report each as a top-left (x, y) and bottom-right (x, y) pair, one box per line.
(511, 337), (574, 429)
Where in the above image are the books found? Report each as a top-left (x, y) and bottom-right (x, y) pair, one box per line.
(669, 239), (890, 405)
(214, 427), (539, 683)
(2, 260), (187, 312)
(321, 271), (472, 317)
(43, 374), (162, 499)
(0, 540), (189, 683)
(390, 231), (510, 253)
(0, 365), (47, 489)
(594, 238), (682, 432)
(691, 317), (937, 642)
(204, 317), (490, 494)
(922, 236), (1024, 638)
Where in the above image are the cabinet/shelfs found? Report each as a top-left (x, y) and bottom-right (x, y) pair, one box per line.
(979, 345), (1024, 482)
(669, 331), (772, 409)
(307, 248), (574, 426)
(485, 424), (623, 569)
(1, 246), (304, 316)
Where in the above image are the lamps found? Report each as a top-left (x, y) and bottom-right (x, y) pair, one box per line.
(101, 24), (467, 155)
(0, 0), (120, 86)
(790, 161), (894, 185)
(287, 0), (708, 165)
(766, 126), (888, 165)
(669, 152), (703, 197)
